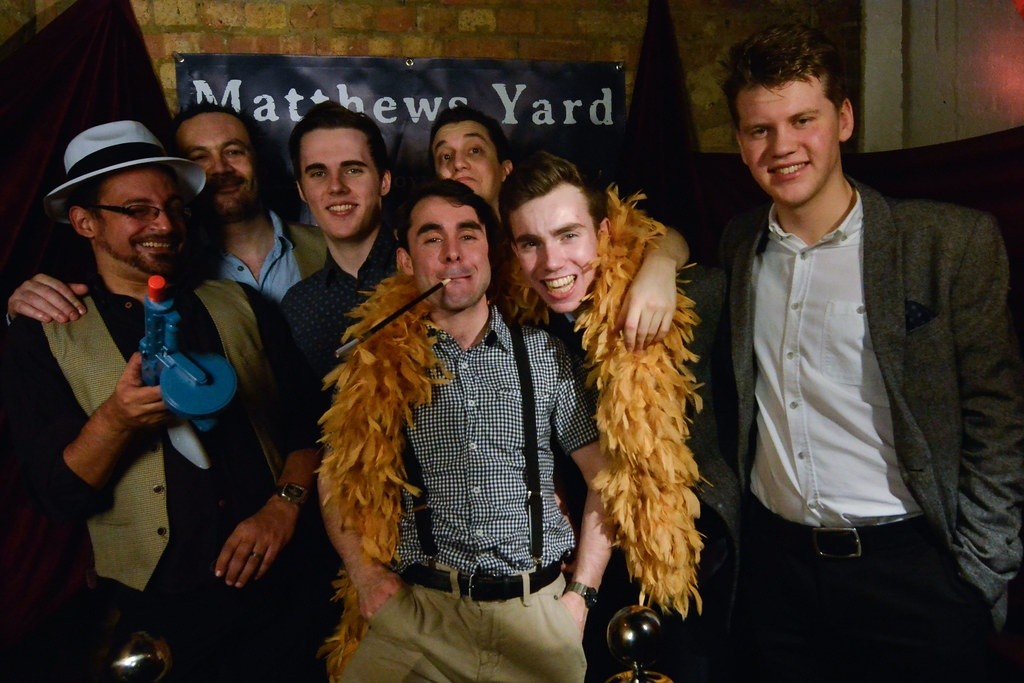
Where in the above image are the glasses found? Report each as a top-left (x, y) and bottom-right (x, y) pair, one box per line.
(85, 205), (193, 225)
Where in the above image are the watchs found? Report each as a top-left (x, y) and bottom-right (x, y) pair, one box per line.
(563, 582), (599, 608)
(272, 481), (308, 511)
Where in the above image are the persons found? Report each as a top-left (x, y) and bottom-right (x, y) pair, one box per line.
(1, 99), (730, 683)
(698, 24), (1024, 683)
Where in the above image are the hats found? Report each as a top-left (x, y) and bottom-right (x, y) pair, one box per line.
(44, 120), (208, 224)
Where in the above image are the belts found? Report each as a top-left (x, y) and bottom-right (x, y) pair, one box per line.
(400, 560), (562, 602)
(744, 493), (932, 557)
